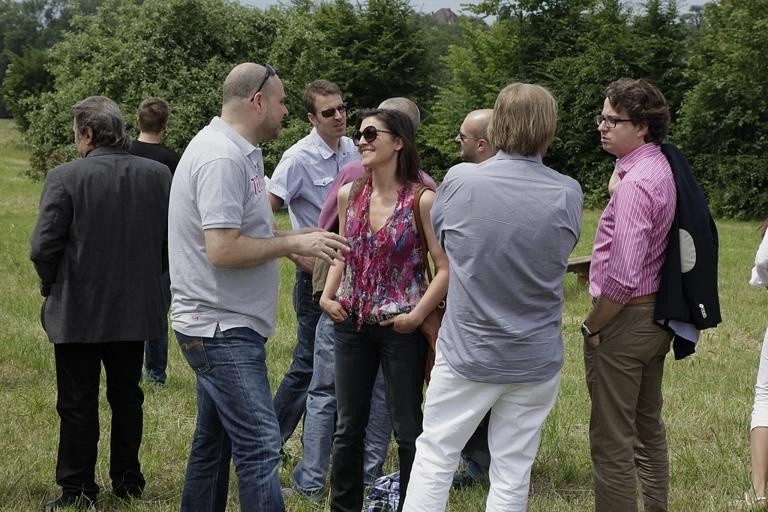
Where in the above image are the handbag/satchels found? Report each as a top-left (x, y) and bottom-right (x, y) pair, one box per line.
(416, 288), (446, 387)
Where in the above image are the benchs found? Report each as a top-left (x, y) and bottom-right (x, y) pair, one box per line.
(565, 254), (592, 285)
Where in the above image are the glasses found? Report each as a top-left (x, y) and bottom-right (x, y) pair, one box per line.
(251, 63), (277, 101)
(353, 126), (398, 146)
(322, 102), (348, 117)
(596, 115), (632, 127)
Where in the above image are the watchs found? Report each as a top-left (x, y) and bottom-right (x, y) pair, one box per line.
(579, 320), (601, 338)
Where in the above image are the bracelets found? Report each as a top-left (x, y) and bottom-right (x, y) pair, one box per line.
(750, 496), (766, 504)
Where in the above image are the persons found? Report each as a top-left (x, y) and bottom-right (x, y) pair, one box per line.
(270, 79), (360, 461)
(288, 97), (499, 512)
(579, 77), (679, 512)
(748, 219), (768, 291)
(28, 95), (172, 506)
(168, 59), (351, 512)
(743, 328), (768, 511)
(119, 96), (183, 385)
(400, 82), (582, 511)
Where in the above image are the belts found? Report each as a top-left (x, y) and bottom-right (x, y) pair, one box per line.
(628, 293), (655, 304)
